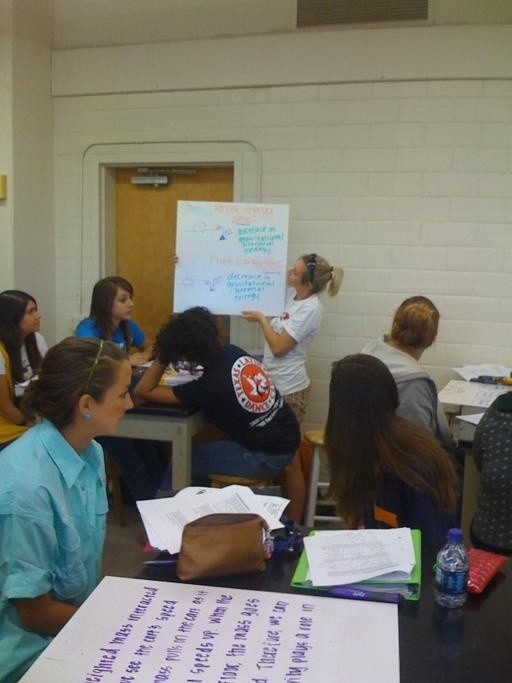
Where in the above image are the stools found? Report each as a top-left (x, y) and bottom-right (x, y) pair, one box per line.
(304, 425), (343, 527)
(208, 470), (274, 493)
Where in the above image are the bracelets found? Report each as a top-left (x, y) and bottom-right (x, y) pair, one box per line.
(151, 361), (162, 368)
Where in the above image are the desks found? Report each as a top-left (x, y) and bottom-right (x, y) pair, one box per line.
(5, 518), (512, 682)
(94, 349), (265, 495)
(455, 366), (512, 550)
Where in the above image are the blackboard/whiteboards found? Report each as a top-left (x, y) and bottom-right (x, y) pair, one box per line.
(171, 199), (290, 318)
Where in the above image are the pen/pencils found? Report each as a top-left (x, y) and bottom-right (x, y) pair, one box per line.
(327, 586), (406, 606)
(142, 559), (176, 566)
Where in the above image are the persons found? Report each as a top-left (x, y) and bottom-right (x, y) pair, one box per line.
(173, 242), (343, 525)
(0, 290), (51, 452)
(133, 306), (300, 493)
(323, 354), (461, 546)
(470, 391), (511, 558)
(356, 294), (465, 489)
(74, 275), (160, 516)
(0, 335), (135, 683)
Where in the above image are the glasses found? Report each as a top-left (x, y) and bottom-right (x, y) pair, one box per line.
(307, 252), (318, 287)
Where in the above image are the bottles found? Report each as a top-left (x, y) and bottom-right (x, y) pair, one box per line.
(438, 529), (471, 610)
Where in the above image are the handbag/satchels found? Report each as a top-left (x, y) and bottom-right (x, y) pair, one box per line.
(177, 513), (269, 582)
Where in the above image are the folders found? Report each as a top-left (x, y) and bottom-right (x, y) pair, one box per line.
(288, 527), (424, 602)
